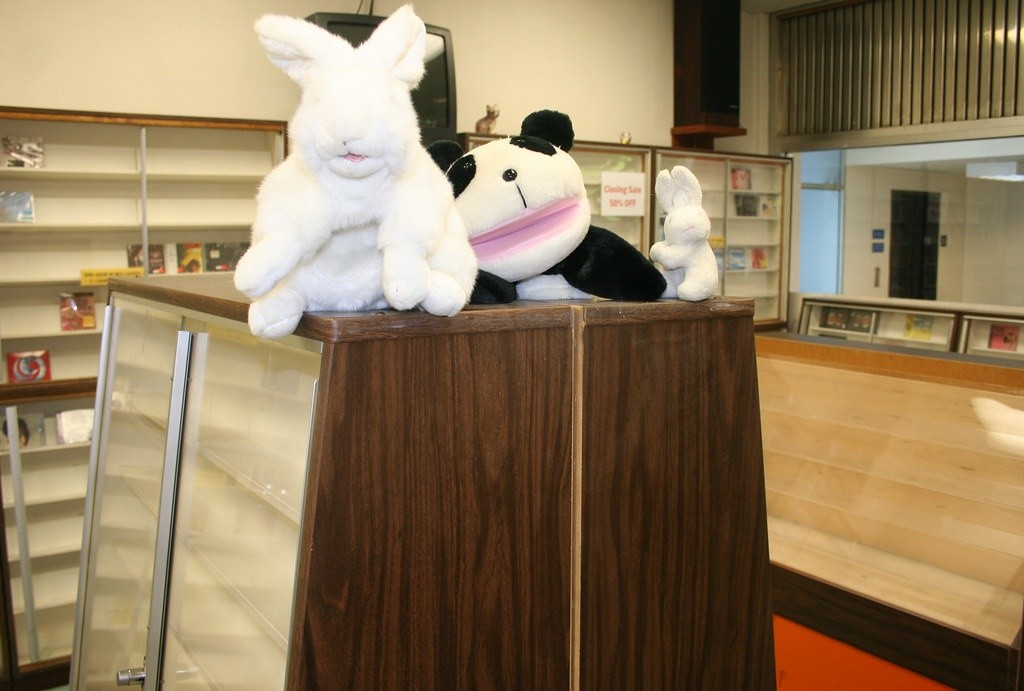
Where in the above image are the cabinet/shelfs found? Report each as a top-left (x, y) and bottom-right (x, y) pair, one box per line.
(0, 102), (792, 691)
(755, 331), (1024, 691)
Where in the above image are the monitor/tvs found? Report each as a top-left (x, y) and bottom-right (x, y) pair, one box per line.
(302, 11), (457, 151)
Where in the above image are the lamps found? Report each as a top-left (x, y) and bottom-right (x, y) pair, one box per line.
(966, 162), (1024, 182)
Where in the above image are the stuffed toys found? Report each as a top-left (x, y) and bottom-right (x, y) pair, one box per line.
(234, 4), (478, 340)
(425, 109), (670, 304)
(476, 104), (500, 134)
(649, 165), (719, 301)
